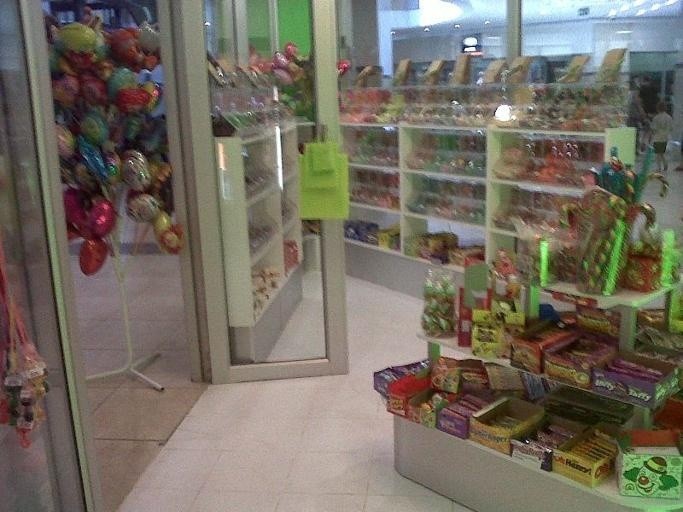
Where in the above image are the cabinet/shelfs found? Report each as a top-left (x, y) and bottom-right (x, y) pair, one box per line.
(212, 121), (301, 328)
(387, 279), (683, 512)
(299, 84), (639, 302)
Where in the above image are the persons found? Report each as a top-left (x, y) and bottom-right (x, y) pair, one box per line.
(628, 94), (649, 156)
(649, 102), (672, 173)
